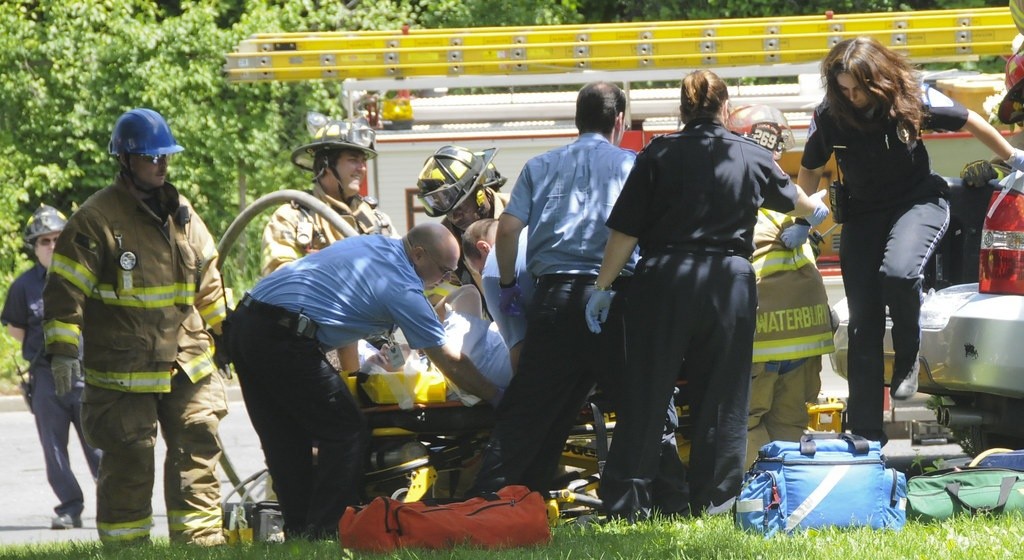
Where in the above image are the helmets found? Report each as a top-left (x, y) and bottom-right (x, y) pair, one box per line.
(728, 105), (795, 160)
(416, 144), (500, 217)
(22, 202), (68, 242)
(290, 120), (378, 172)
(998, 50), (1024, 124)
(108, 108), (185, 155)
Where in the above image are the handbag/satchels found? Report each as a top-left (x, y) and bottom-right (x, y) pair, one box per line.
(733, 432), (908, 538)
(907, 467), (1024, 524)
(338, 484), (552, 553)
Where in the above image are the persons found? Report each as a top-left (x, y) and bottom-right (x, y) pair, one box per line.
(780, 38), (1024, 449)
(959, 0), (1024, 188)
(223, 222), (506, 544)
(463, 82), (692, 515)
(0, 202), (103, 530)
(258, 102), (841, 529)
(43, 109), (237, 550)
(584, 69), (831, 527)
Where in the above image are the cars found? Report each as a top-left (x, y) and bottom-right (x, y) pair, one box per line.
(824, 172), (1024, 403)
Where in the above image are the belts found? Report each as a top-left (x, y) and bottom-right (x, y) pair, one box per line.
(241, 294), (318, 338)
(542, 274), (595, 284)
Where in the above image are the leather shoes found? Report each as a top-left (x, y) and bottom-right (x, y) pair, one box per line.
(51, 512), (83, 529)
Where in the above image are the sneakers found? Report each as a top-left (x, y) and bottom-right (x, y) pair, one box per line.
(890, 352), (920, 400)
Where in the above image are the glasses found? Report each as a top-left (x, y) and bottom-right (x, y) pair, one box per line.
(334, 128), (376, 149)
(417, 157), (483, 216)
(424, 250), (452, 276)
(136, 155), (170, 164)
(36, 237), (58, 246)
(625, 118), (630, 132)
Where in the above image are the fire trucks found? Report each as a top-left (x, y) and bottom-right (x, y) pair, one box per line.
(217, 6), (1024, 300)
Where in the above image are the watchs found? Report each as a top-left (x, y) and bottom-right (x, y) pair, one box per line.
(594, 281), (612, 291)
(1003, 147), (1016, 164)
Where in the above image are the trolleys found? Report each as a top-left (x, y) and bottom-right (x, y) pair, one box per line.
(360, 382), (695, 528)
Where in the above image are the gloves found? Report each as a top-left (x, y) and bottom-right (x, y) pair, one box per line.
(499, 284), (522, 316)
(960, 160), (999, 187)
(806, 188), (829, 226)
(585, 291), (611, 333)
(780, 224), (810, 250)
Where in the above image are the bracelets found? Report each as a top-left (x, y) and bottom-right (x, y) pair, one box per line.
(795, 218), (811, 227)
(498, 277), (517, 289)
